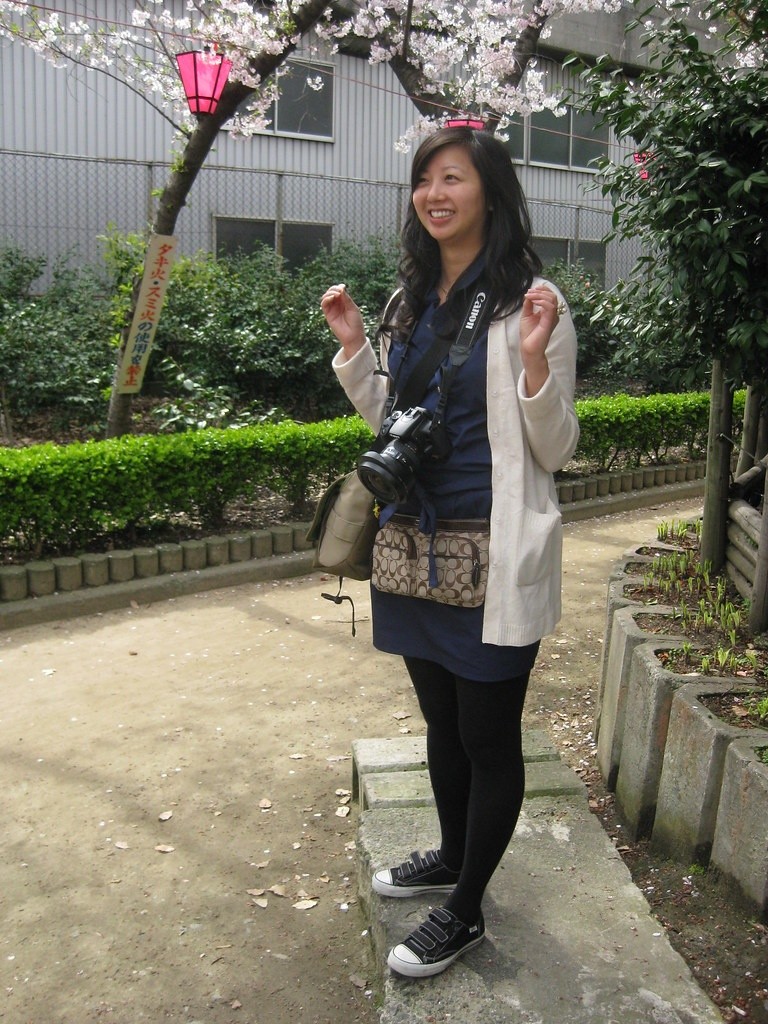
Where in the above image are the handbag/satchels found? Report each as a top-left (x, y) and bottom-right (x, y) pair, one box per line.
(371, 510), (490, 609)
(305, 469), (376, 581)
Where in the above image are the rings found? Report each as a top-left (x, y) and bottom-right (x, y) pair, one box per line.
(555, 302), (568, 316)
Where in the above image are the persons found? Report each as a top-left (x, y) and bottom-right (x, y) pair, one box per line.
(318, 126), (581, 978)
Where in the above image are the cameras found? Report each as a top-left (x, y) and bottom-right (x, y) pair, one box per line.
(357, 405), (452, 504)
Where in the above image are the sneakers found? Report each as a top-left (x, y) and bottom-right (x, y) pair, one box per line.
(372, 850), (458, 898)
(387, 904), (485, 976)
